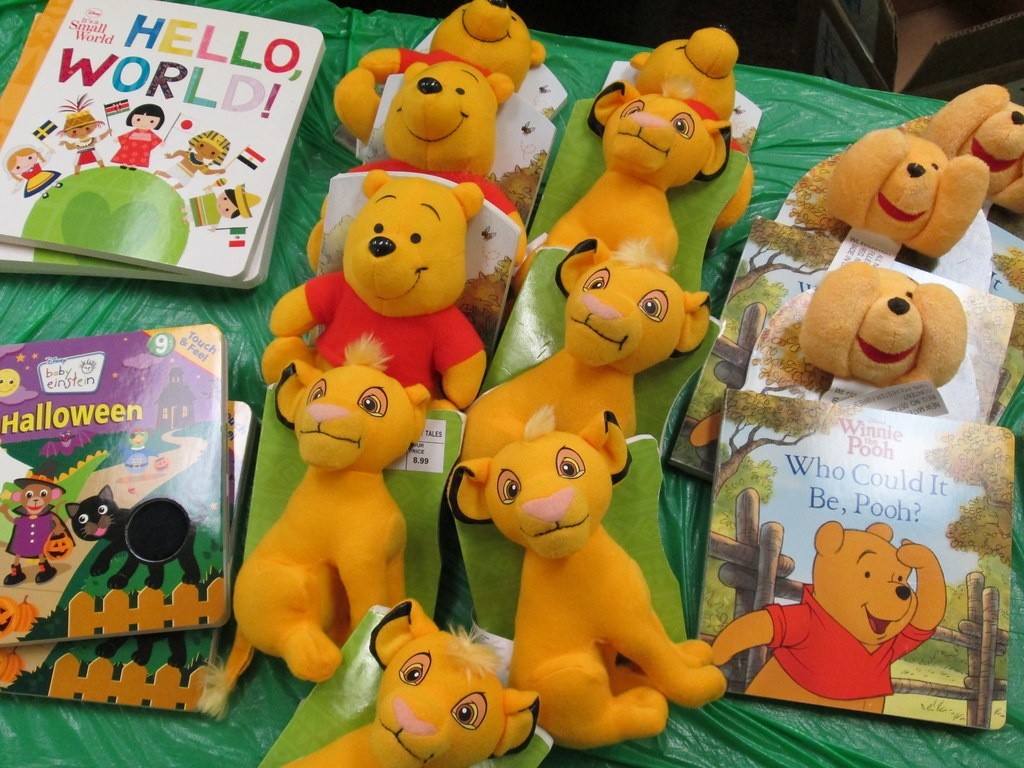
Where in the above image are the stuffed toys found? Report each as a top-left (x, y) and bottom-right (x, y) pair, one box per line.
(824, 83), (1023, 258)
(798, 262), (967, 389)
(198, 1), (753, 767)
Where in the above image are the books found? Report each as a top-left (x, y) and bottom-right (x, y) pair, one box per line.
(668, 215), (1023, 731)
(0, 0), (326, 290)
(1, 324), (254, 713)
